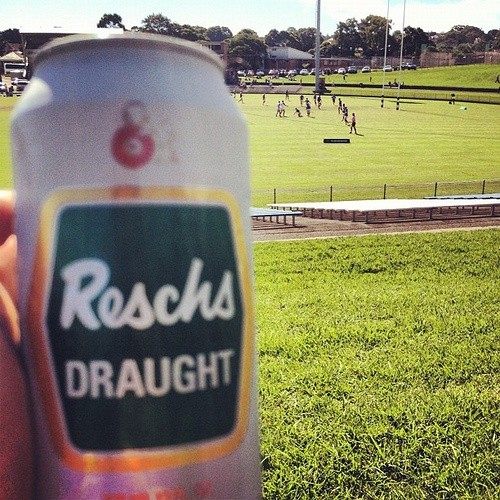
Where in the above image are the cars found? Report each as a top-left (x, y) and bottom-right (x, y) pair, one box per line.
(362, 66), (371, 73)
(268, 67), (309, 75)
(237, 68), (265, 76)
(384, 65), (392, 72)
(311, 67), (331, 75)
(332, 66), (357, 74)
(392, 62), (416, 70)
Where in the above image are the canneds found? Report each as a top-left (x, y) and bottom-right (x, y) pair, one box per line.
(9, 29), (262, 500)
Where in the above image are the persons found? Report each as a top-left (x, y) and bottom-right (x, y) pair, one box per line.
(331, 94), (348, 122)
(0, 190), (35, 500)
(263, 93), (265, 104)
(233, 89), (243, 104)
(495, 75), (500, 83)
(300, 93), (311, 116)
(286, 90), (289, 99)
(294, 108), (303, 117)
(313, 93), (321, 109)
(276, 100), (286, 117)
(349, 113), (357, 134)
